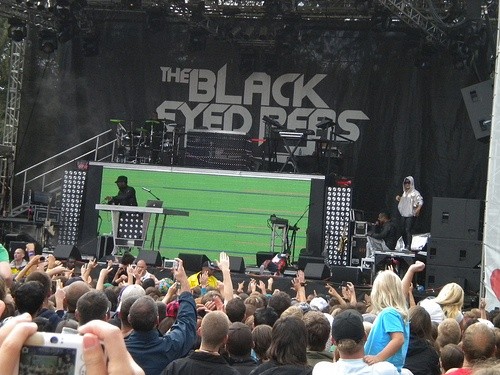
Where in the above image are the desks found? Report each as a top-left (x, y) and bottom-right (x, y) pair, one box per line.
(95, 203), (189, 255)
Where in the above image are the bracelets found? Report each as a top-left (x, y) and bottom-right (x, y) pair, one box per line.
(300, 283), (307, 286)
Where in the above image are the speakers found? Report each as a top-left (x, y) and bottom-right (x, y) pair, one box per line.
(178, 253), (211, 273)
(461, 79), (494, 140)
(228, 256), (246, 272)
(256, 251), (276, 268)
(304, 262), (330, 280)
(297, 256), (324, 271)
(53, 244), (81, 260)
(135, 249), (163, 267)
(331, 266), (363, 284)
(5, 230), (43, 255)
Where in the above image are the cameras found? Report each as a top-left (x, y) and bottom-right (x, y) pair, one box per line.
(17, 330), (87, 375)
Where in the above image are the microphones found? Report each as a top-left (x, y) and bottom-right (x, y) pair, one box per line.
(309, 202), (316, 206)
(142, 186), (160, 200)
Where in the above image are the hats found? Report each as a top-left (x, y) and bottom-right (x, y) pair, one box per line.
(103, 283), (114, 289)
(310, 297), (329, 310)
(121, 284), (146, 301)
(201, 260), (218, 268)
(332, 308), (364, 342)
(115, 176), (128, 184)
(165, 300), (179, 317)
(159, 278), (174, 294)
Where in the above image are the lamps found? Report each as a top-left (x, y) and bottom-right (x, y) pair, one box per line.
(79, 35), (100, 56)
(38, 30), (59, 54)
(242, 22), (254, 40)
(7, 17), (27, 42)
(257, 25), (268, 40)
(226, 25), (241, 38)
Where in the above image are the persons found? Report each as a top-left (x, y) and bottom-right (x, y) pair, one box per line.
(105, 175), (138, 254)
(365, 210), (398, 258)
(0, 243), (500, 375)
(396, 176), (424, 252)
